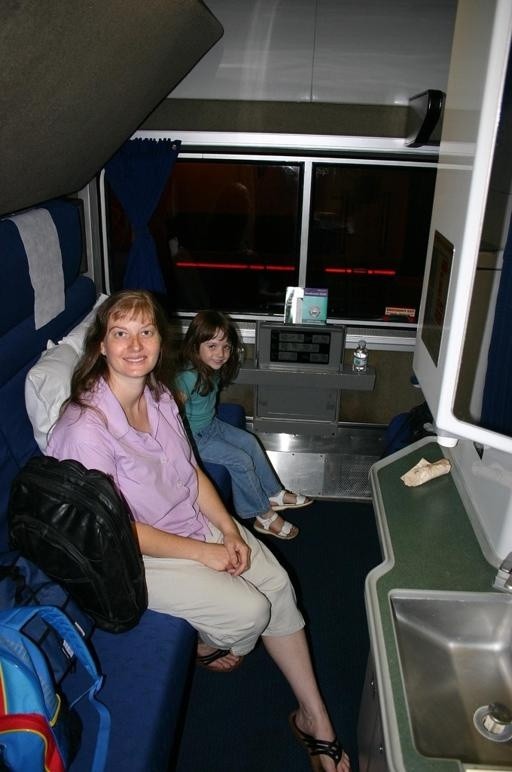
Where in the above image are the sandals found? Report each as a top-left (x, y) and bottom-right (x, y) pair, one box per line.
(253, 512), (299, 540)
(268, 489), (313, 512)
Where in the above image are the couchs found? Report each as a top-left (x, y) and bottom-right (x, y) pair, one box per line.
(0, 197), (246, 772)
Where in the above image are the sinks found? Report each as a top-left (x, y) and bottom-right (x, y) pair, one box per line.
(383, 585), (512, 771)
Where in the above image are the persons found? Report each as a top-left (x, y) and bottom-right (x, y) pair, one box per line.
(46, 288), (349, 772)
(163, 311), (313, 539)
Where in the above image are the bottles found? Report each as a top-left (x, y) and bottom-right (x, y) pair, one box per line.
(352, 340), (368, 373)
(237, 336), (245, 367)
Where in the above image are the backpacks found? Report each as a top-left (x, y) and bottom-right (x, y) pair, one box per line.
(0, 604), (113, 772)
(3, 454), (149, 635)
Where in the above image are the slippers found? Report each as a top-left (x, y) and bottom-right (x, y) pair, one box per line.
(288, 708), (350, 772)
(192, 648), (245, 674)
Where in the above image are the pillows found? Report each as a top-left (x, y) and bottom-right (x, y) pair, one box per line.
(24, 292), (109, 457)
